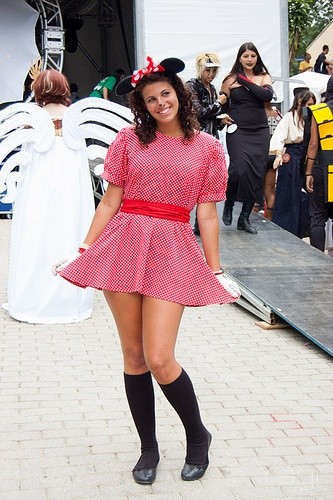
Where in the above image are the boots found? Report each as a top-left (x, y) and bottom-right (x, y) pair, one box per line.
(237, 200), (257, 234)
(222, 197), (237, 226)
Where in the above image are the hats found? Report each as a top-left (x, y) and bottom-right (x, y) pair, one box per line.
(195, 52), (222, 68)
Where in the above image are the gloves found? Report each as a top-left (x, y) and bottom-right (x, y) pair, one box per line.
(53, 243), (92, 277)
(237, 71), (273, 102)
(213, 270), (242, 303)
(219, 91), (229, 114)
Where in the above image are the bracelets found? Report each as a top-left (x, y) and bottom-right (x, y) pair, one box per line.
(305, 174), (313, 177)
(276, 154), (282, 158)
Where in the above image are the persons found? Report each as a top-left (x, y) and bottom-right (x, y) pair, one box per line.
(314, 45), (333, 74)
(252, 75), (333, 253)
(299, 53), (313, 73)
(8, 69), (96, 324)
(184, 53), (234, 236)
(67, 83), (81, 107)
(88, 69), (126, 148)
(221, 42), (273, 234)
(54, 57), (241, 485)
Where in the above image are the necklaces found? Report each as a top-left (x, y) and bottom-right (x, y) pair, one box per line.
(250, 74), (254, 80)
(204, 84), (209, 91)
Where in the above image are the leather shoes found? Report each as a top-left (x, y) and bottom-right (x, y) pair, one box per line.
(132, 442), (160, 484)
(180, 429), (213, 481)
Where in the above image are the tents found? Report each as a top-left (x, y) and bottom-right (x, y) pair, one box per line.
(269, 70), (333, 116)
